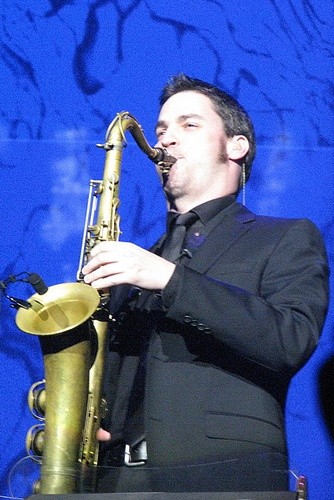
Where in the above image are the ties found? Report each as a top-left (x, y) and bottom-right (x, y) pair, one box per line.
(111, 214), (198, 430)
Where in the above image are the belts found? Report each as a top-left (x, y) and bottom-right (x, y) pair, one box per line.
(99, 440), (149, 470)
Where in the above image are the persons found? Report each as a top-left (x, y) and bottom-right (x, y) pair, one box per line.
(82, 73), (330, 499)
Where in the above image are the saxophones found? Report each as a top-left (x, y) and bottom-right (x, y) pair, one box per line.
(13, 110), (179, 496)
